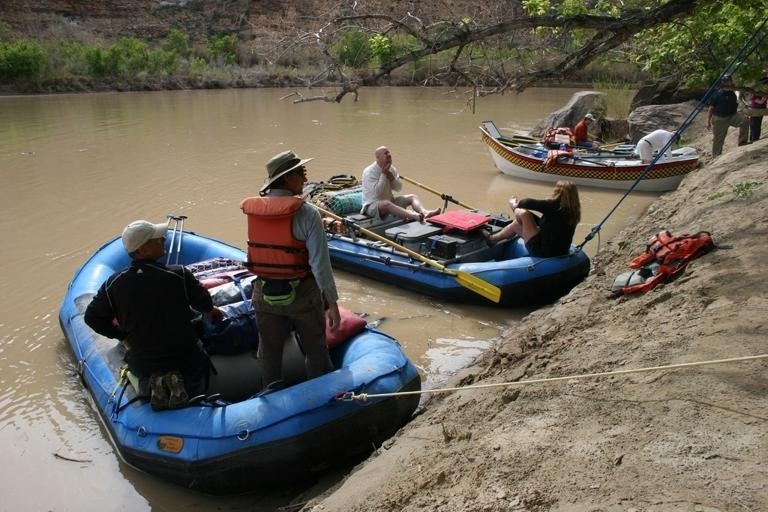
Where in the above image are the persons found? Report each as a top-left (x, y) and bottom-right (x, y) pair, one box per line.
(705, 73), (750, 160)
(626, 129), (682, 164)
(575, 113), (600, 147)
(480, 178), (580, 258)
(239, 148), (341, 394)
(82, 219), (223, 402)
(359, 147), (442, 221)
(750, 79), (768, 143)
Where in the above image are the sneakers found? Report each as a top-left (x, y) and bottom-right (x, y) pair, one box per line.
(150, 374), (169, 411)
(481, 229), (495, 247)
(166, 372), (187, 409)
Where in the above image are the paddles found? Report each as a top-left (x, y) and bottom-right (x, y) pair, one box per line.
(513, 136), (633, 154)
(305, 203), (501, 305)
(499, 141), (610, 169)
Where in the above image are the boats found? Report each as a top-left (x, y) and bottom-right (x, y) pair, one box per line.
(301, 180), (591, 309)
(478, 124), (700, 193)
(56, 226), (422, 499)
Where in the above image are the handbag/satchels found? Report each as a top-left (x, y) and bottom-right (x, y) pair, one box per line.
(258, 279), (300, 306)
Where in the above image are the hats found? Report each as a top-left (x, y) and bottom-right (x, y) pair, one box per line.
(260, 149), (314, 193)
(585, 113), (596, 121)
(122, 220), (169, 253)
